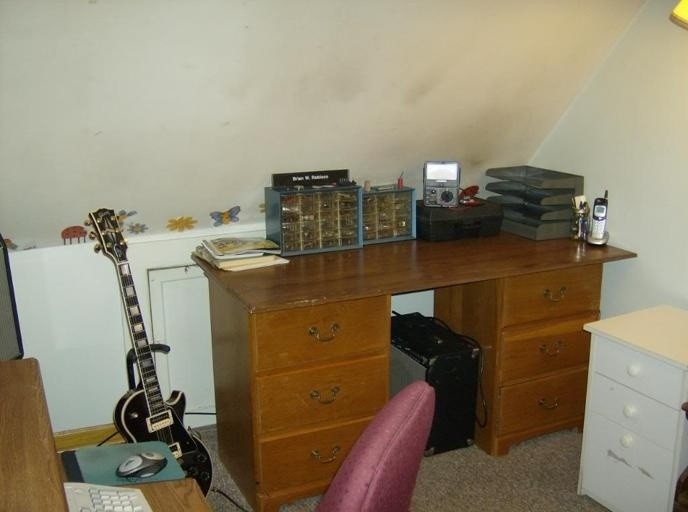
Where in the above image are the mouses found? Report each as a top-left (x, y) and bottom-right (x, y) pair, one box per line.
(116, 453), (167, 479)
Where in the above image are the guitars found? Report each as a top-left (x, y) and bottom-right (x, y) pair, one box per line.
(85, 208), (214, 501)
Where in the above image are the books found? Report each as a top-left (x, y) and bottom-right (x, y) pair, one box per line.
(190, 237), (291, 272)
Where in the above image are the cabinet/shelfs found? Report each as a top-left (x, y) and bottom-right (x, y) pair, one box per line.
(191, 230), (637, 512)
(576, 304), (688, 512)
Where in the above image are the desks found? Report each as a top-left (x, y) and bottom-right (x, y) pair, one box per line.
(0, 357), (211, 512)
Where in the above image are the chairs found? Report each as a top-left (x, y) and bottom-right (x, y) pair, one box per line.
(317, 379), (436, 512)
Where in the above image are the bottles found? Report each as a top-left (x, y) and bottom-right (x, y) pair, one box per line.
(568, 208), (590, 242)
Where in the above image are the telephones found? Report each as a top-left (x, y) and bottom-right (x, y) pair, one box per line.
(586, 190), (609, 246)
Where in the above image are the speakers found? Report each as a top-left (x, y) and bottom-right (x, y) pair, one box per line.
(390, 311), (479, 457)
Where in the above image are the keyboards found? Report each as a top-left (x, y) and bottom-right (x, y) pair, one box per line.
(63, 482), (153, 512)
